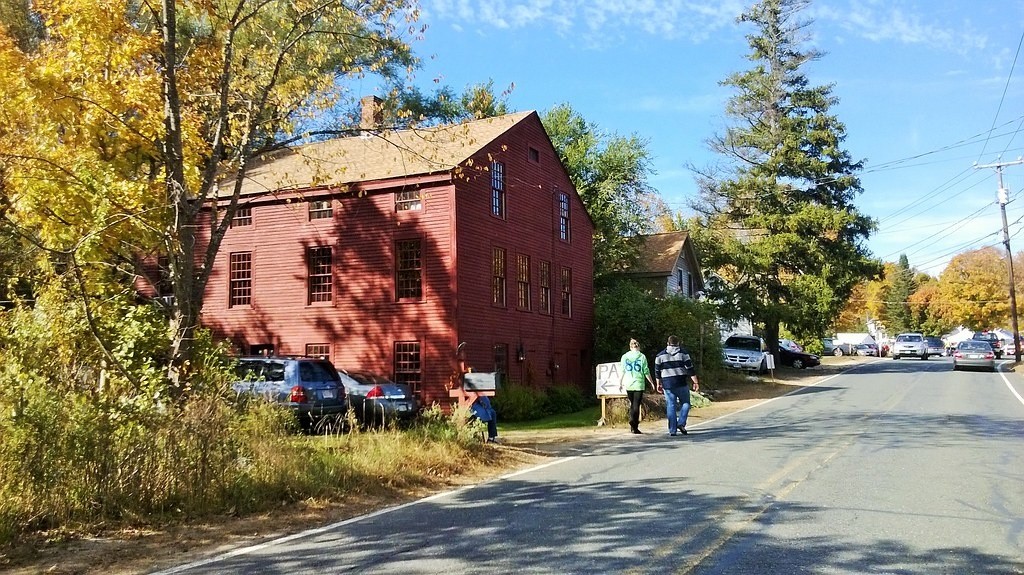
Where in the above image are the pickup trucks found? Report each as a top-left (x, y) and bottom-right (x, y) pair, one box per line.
(971, 330), (1005, 358)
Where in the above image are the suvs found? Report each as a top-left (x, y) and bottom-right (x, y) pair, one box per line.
(721, 333), (769, 376)
(226, 356), (348, 421)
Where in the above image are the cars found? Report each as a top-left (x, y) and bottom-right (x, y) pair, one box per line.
(923, 336), (947, 357)
(951, 341), (996, 372)
(893, 333), (929, 360)
(337, 367), (418, 419)
(778, 337), (894, 369)
(999, 338), (1024, 353)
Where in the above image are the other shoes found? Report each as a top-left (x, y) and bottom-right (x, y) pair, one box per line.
(677, 423), (687, 435)
(629, 420), (641, 434)
(487, 437), (499, 444)
(671, 432), (677, 436)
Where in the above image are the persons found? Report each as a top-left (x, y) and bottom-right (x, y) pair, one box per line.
(655, 335), (698, 436)
(618, 338), (655, 434)
